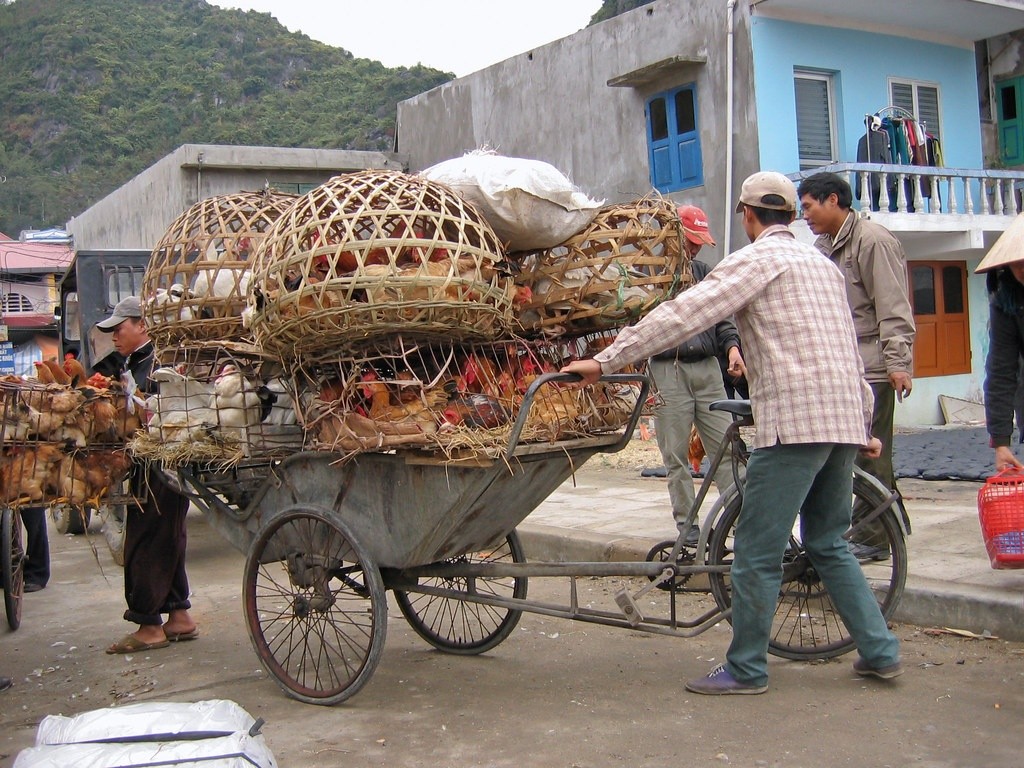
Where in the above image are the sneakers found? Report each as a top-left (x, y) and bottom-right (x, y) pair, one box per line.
(685, 662), (768, 695)
(23, 583), (42, 593)
(853, 657), (904, 678)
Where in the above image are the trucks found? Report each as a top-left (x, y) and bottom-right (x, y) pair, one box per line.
(54, 247), (249, 390)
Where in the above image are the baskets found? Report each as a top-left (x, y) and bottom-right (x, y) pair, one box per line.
(977, 466), (1024, 569)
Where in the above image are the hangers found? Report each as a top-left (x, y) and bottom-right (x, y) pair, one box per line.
(864, 106), (936, 141)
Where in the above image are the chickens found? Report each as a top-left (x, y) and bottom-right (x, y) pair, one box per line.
(0, 207), (707, 514)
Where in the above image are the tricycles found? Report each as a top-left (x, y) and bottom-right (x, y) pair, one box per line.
(175, 366), (913, 706)
(0, 503), (29, 630)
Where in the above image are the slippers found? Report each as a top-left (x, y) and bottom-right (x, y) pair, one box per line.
(163, 626), (199, 641)
(105, 634), (170, 654)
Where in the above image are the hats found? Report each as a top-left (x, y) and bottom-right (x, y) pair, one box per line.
(735, 172), (796, 213)
(973, 211), (1024, 274)
(676, 205), (716, 248)
(96, 296), (141, 333)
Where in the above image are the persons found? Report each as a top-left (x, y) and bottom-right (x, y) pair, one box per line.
(96, 297), (200, 654)
(10, 507), (50, 593)
(560, 171), (906, 696)
(983, 246), (1024, 474)
(622, 206), (751, 555)
(798, 175), (917, 560)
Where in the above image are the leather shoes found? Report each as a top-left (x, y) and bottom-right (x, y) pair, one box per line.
(684, 525), (700, 544)
(846, 540), (889, 560)
